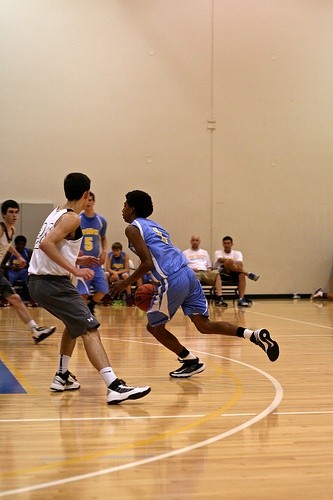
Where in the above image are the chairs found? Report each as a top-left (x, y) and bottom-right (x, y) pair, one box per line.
(13, 260), (239, 300)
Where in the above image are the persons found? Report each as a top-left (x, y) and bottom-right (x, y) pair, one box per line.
(182, 234), (228, 306)
(103, 241), (135, 307)
(310, 288), (333, 300)
(0, 200), (56, 344)
(28, 173), (151, 405)
(76, 191), (109, 314)
(136, 270), (161, 286)
(110, 190), (280, 379)
(214, 236), (260, 307)
(4, 234), (39, 306)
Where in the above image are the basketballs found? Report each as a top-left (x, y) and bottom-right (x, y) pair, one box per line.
(134, 284), (156, 311)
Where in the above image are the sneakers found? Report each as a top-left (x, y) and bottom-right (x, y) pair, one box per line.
(106, 407), (148, 419)
(126, 300), (135, 306)
(215, 301), (228, 307)
(104, 300), (113, 306)
(255, 329), (279, 362)
(31, 300), (38, 307)
(1, 301), (10, 306)
(248, 272), (259, 281)
(106, 379), (151, 404)
(48, 392), (80, 407)
(238, 298), (248, 306)
(168, 358), (206, 378)
(32, 325), (55, 344)
(50, 371), (81, 392)
(169, 378), (207, 391)
